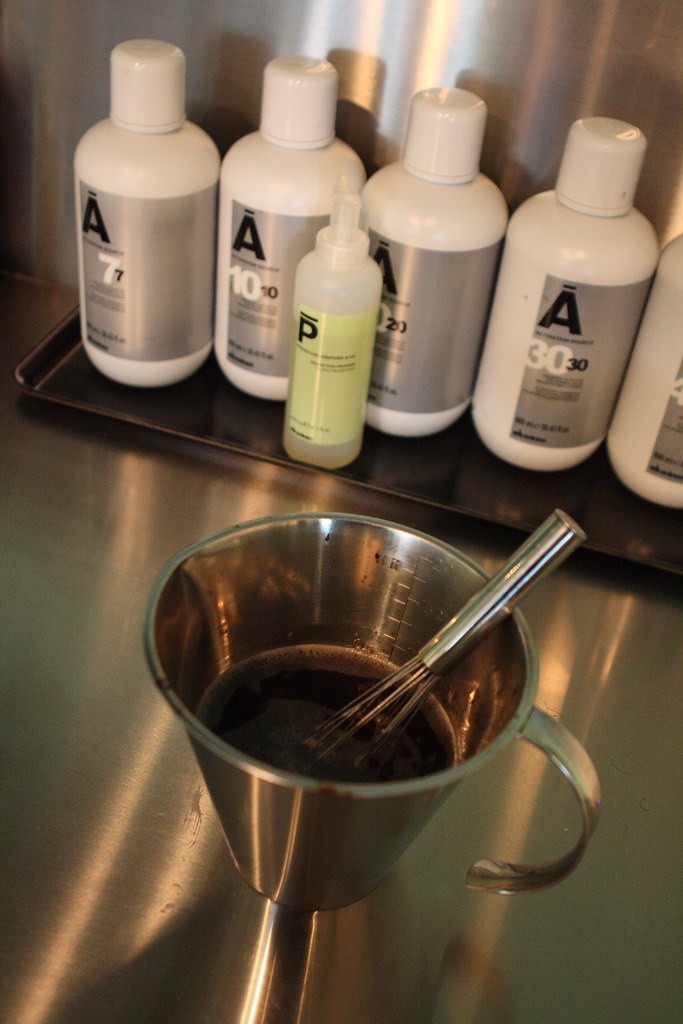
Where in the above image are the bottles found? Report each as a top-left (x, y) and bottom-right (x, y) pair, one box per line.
(73, 39), (220, 388)
(282, 180), (383, 469)
(213, 55), (366, 400)
(359, 86), (508, 437)
(471, 116), (661, 472)
(606, 232), (683, 510)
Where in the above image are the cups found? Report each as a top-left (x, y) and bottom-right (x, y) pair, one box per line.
(143, 510), (600, 911)
